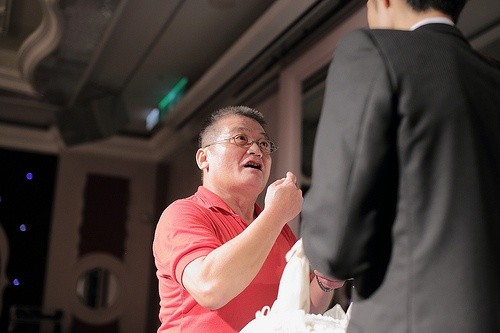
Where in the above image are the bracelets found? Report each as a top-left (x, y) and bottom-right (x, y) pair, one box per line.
(315, 275), (333, 293)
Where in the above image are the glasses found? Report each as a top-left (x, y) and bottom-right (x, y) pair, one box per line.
(203, 134), (279, 154)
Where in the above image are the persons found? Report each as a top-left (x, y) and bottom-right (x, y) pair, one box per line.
(302, 0), (500, 333)
(152, 107), (345, 333)
